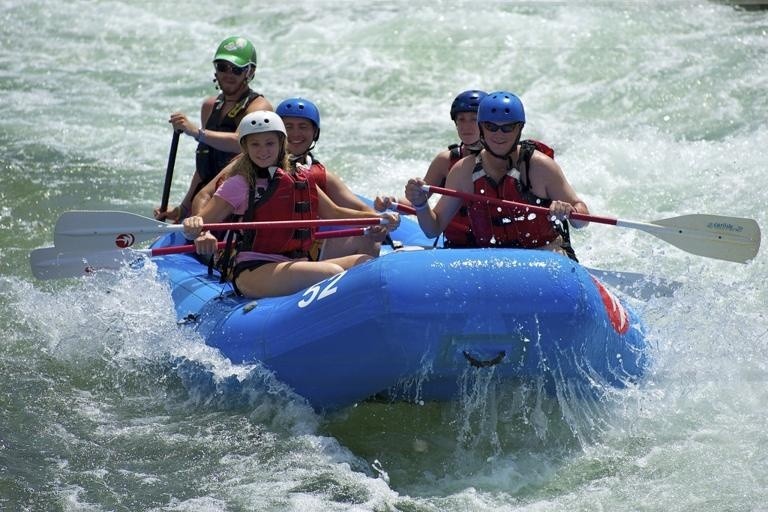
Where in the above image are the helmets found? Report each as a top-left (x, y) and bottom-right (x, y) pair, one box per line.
(476, 91), (526, 127)
(450, 90), (487, 120)
(276, 98), (320, 129)
(236, 111), (288, 144)
(212, 36), (258, 67)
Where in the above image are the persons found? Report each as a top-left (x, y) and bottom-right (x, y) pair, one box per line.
(374, 89), (489, 252)
(183, 110), (401, 299)
(153, 37), (273, 221)
(192, 99), (387, 278)
(405, 92), (589, 261)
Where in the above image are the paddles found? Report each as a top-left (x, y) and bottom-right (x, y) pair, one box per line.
(420, 183), (760, 265)
(54, 210), (391, 255)
(30, 229), (370, 281)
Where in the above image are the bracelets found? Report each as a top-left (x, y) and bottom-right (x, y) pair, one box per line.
(573, 207), (578, 212)
(195, 128), (205, 143)
(412, 196), (428, 210)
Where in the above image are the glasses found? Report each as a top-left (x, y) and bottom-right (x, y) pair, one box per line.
(214, 62), (248, 76)
(483, 120), (519, 132)
